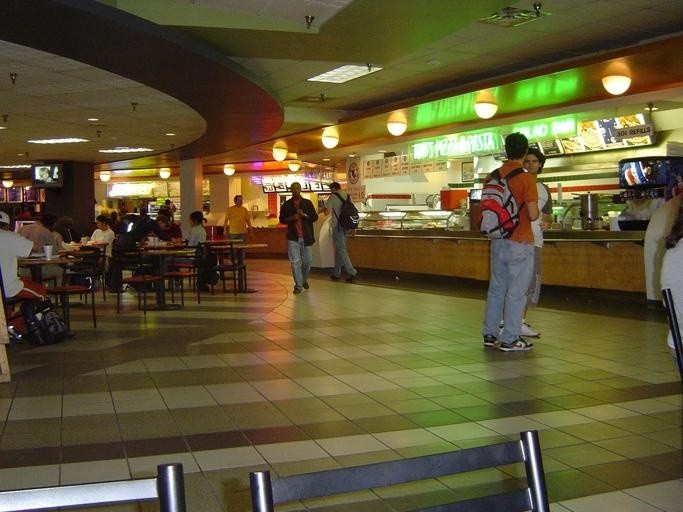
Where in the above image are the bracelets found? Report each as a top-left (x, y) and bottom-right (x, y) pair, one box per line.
(303, 214), (306, 218)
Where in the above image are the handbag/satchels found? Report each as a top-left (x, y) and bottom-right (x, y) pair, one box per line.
(117, 232), (137, 252)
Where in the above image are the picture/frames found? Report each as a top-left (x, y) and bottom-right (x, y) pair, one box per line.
(0, 187), (7, 204)
(37, 187), (47, 203)
(23, 185), (39, 204)
(7, 186), (23, 204)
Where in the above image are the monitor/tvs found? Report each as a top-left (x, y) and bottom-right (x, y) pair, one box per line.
(31, 163), (65, 188)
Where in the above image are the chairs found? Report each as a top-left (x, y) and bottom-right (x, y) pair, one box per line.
(660, 287), (683, 389)
(1, 230), (249, 347)
(0, 461), (186, 512)
(248, 429), (550, 512)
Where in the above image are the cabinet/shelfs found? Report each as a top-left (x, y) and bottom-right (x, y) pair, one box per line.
(358, 209), (466, 231)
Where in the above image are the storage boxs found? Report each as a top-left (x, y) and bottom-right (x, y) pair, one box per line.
(440, 189), (469, 211)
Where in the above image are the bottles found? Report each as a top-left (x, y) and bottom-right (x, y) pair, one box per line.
(587, 214), (609, 230)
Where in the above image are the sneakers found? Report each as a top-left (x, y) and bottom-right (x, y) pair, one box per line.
(293, 285), (303, 294)
(345, 272), (360, 282)
(498, 319), (504, 335)
(7, 325), (23, 342)
(498, 337), (535, 352)
(303, 282), (310, 289)
(483, 333), (499, 346)
(175, 278), (181, 289)
(520, 320), (540, 337)
(331, 274), (341, 280)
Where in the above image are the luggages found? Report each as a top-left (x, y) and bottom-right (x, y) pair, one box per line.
(131, 263), (155, 292)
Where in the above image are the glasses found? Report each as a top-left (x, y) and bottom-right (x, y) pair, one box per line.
(523, 159), (540, 163)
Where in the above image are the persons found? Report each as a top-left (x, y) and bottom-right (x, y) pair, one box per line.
(54, 216), (73, 242)
(18, 213), (64, 287)
(172, 211), (206, 269)
(480, 132), (539, 352)
(0, 211), (47, 341)
(279, 182), (318, 293)
(140, 215), (172, 261)
(223, 195), (255, 264)
(324, 182), (358, 282)
(39, 168), (53, 183)
(644, 168), (683, 372)
(499, 149), (553, 337)
(158, 200), (174, 222)
(90, 215), (115, 257)
(112, 208), (153, 252)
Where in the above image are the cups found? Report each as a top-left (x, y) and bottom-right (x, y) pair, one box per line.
(44, 245), (53, 262)
(81, 237), (87, 247)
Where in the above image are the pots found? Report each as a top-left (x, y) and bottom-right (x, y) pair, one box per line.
(579, 192), (598, 230)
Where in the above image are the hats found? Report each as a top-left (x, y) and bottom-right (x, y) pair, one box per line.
(0, 211), (10, 225)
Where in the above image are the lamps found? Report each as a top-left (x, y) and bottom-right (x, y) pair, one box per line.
(99, 170), (112, 183)
(386, 112), (409, 137)
(288, 160), (301, 172)
(473, 93), (499, 121)
(272, 140), (289, 162)
(159, 167), (172, 179)
(223, 164), (236, 177)
(2, 179), (14, 188)
(600, 61), (634, 97)
(321, 127), (340, 150)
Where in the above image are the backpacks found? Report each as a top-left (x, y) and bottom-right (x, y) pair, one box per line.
(159, 206), (170, 222)
(332, 191), (359, 229)
(20, 296), (69, 346)
(203, 252), (220, 286)
(104, 264), (123, 293)
(476, 167), (525, 240)
